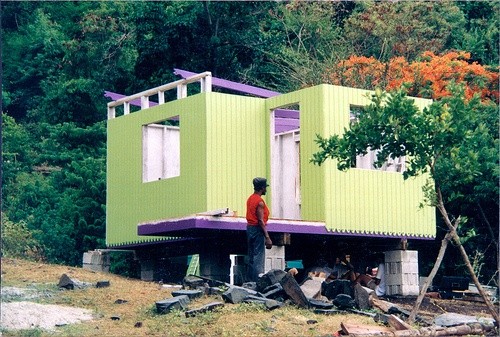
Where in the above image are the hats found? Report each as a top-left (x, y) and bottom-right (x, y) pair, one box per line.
(253, 178), (269, 187)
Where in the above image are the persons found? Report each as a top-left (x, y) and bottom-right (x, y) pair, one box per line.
(325, 252), (358, 285)
(357, 253), (388, 296)
(245, 177), (273, 285)
